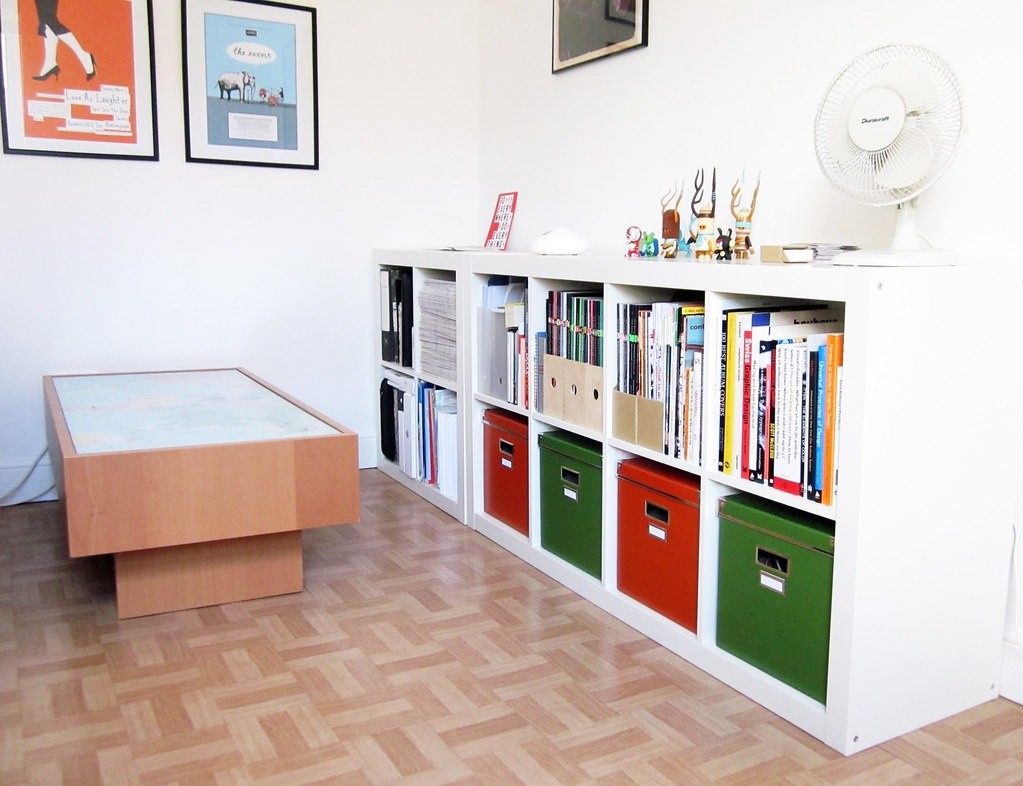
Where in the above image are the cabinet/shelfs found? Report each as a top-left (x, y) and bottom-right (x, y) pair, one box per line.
(460, 251), (998, 757)
(369, 248), (529, 526)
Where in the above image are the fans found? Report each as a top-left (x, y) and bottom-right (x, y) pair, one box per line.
(812, 41), (968, 266)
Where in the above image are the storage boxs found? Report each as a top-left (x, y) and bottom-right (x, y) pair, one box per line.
(618, 458), (700, 635)
(537, 430), (602, 580)
(716, 493), (833, 707)
(481, 408), (529, 538)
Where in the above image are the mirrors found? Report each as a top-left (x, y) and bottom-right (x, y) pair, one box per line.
(552, 0), (648, 74)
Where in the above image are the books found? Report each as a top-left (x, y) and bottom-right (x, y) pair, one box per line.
(507, 288), (530, 411)
(718, 303), (844, 509)
(617, 301), (704, 466)
(534, 289), (604, 413)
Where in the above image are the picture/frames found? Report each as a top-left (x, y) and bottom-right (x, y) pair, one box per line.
(0, 0), (160, 162)
(181, 0), (320, 170)
